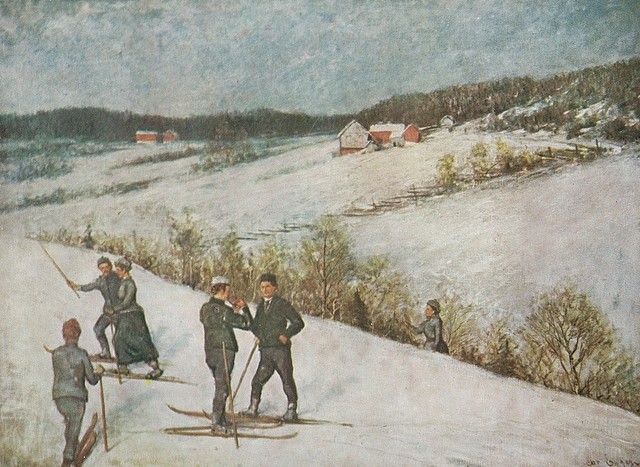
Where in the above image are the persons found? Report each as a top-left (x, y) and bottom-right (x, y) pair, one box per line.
(401, 298), (450, 354)
(105, 259), (162, 381)
(68, 257), (123, 359)
(240, 274), (305, 420)
(51, 319), (105, 462)
(199, 276), (253, 435)
